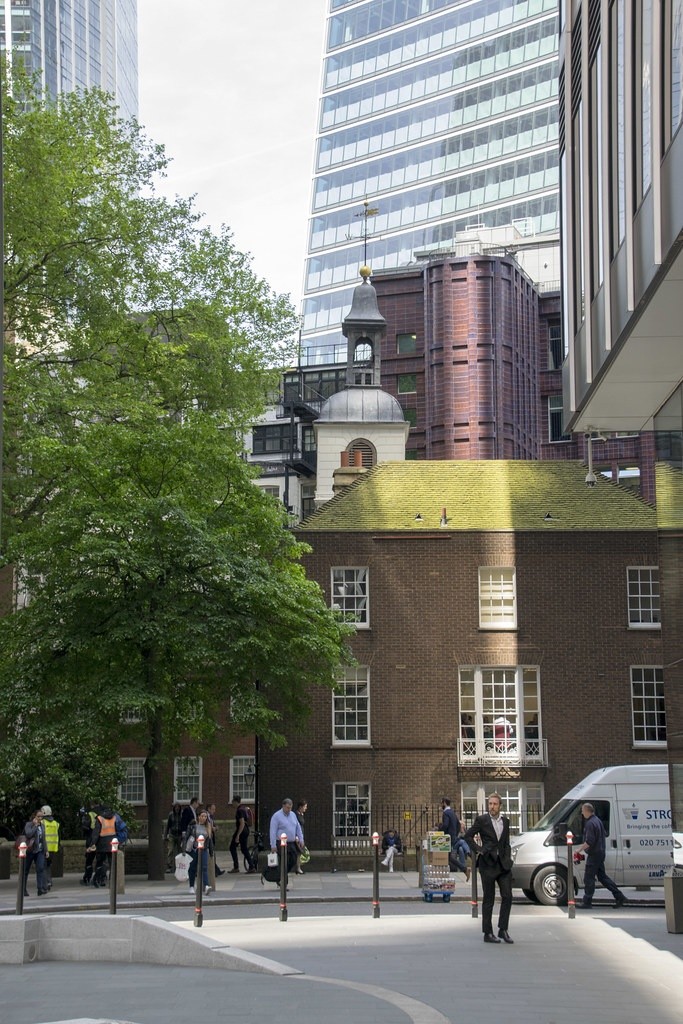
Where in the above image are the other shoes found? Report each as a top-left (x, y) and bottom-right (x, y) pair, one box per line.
(205, 885), (212, 895)
(23, 890), (29, 896)
(215, 870), (225, 877)
(389, 869), (393, 872)
(245, 868), (256, 874)
(188, 887), (194, 894)
(227, 868), (239, 874)
(381, 861), (388, 866)
(576, 902), (592, 909)
(79, 879), (90, 886)
(100, 881), (106, 887)
(37, 888), (47, 896)
(613, 896), (627, 909)
(465, 868), (471, 882)
(93, 877), (100, 888)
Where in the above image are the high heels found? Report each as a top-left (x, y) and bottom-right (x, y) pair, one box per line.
(295, 869), (306, 875)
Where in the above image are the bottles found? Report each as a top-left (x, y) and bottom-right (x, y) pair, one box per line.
(424, 864), (455, 890)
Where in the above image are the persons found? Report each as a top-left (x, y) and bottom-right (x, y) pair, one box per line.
(438, 797), (471, 882)
(269, 798), (303, 892)
(40, 805), (61, 891)
(22, 810), (48, 895)
(205, 804), (226, 877)
(380, 828), (402, 873)
(452, 820), (472, 867)
(462, 713), (538, 739)
(294, 798), (308, 874)
(87, 805), (128, 887)
(183, 810), (215, 896)
(575, 803), (629, 909)
(226, 794), (256, 873)
(165, 803), (183, 873)
(80, 800), (103, 885)
(180, 797), (199, 838)
(464, 794), (516, 944)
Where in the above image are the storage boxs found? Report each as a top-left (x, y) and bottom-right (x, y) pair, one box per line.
(420, 829), (452, 867)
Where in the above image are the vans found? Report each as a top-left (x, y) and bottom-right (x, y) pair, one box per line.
(507, 762), (683, 907)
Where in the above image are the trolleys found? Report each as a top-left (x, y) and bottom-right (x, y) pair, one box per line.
(421, 854), (455, 902)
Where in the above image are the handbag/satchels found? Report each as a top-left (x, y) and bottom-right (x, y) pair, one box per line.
(262, 850), (281, 882)
(174, 853), (193, 882)
(300, 846), (310, 864)
(180, 834), (194, 852)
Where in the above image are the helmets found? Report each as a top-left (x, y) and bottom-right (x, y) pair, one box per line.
(41, 805), (52, 816)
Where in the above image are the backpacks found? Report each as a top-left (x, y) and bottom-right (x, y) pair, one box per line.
(239, 806), (253, 827)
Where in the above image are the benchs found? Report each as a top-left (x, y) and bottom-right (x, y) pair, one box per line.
(330, 833), (407, 873)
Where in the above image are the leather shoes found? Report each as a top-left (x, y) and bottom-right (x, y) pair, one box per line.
(498, 928), (514, 943)
(484, 932), (501, 943)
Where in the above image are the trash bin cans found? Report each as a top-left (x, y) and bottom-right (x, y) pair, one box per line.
(0, 837), (10, 880)
(663, 867), (683, 934)
(50, 843), (64, 878)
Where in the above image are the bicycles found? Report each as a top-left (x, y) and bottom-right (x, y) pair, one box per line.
(243, 830), (279, 874)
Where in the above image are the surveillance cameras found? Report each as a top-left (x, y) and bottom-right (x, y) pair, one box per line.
(586, 472), (598, 487)
